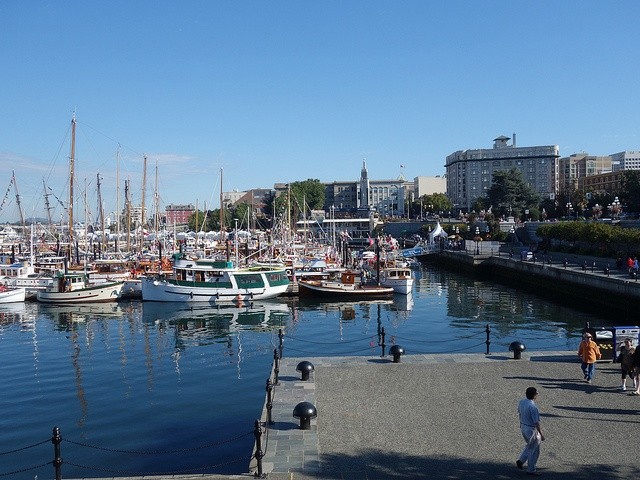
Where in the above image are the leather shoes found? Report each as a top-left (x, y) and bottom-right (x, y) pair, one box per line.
(527, 471), (544, 475)
(516, 460), (524, 470)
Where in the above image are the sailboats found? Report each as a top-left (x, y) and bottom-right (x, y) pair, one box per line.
(87, 145), (143, 283)
(8, 110), (85, 297)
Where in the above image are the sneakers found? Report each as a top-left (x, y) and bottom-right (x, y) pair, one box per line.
(584, 375), (587, 379)
(585, 379), (591, 383)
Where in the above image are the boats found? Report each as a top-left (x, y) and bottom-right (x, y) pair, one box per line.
(142, 255), (289, 300)
(0, 287), (25, 302)
(145, 157), (292, 265)
(381, 267), (413, 293)
(298, 271), (393, 298)
(286, 188), (364, 293)
(38, 282), (124, 302)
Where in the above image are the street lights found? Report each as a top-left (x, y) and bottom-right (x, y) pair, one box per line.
(612, 197), (620, 212)
(566, 203), (574, 222)
(475, 227), (480, 256)
(420, 200), (434, 220)
(510, 226), (516, 251)
(456, 227), (460, 244)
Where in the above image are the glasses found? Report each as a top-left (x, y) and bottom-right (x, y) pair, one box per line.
(625, 340), (631, 343)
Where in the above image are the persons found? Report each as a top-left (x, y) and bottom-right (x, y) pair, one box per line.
(582, 320), (596, 342)
(629, 334), (640, 395)
(578, 332), (602, 383)
(616, 338), (636, 391)
(516, 387), (546, 476)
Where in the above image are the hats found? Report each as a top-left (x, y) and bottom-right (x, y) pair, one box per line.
(585, 333), (591, 338)
